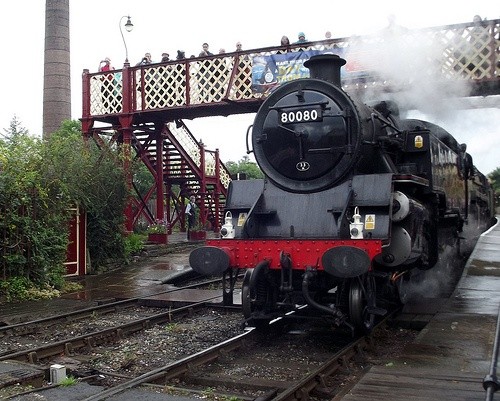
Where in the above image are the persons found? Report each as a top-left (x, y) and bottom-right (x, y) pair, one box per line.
(134, 53), (154, 67)
(198, 43), (214, 57)
(160, 53), (171, 63)
(276, 35), (292, 54)
(184, 195), (197, 240)
(294, 32), (309, 43)
(235, 42), (244, 52)
(98, 57), (116, 115)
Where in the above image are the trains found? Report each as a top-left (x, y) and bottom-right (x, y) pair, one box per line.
(188, 53), (496, 334)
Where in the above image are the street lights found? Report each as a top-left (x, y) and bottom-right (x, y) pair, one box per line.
(119, 13), (134, 263)
